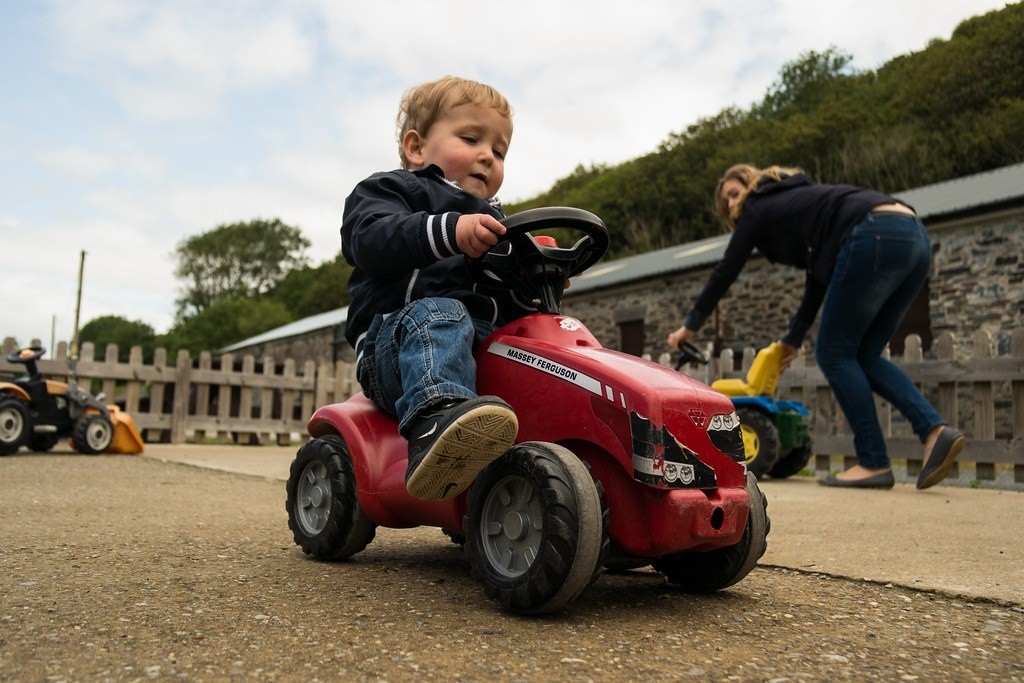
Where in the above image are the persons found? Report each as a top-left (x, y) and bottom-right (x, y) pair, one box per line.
(665, 161), (967, 491)
(339, 75), (584, 504)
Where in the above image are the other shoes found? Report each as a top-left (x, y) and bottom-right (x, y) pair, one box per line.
(816, 469), (895, 490)
(916, 427), (967, 489)
(405, 394), (519, 501)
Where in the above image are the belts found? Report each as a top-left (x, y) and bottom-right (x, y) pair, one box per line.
(872, 211), (921, 223)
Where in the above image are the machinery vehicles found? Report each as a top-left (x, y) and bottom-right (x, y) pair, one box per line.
(0, 347), (144, 456)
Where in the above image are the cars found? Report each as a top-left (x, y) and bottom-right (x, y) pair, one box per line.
(672, 340), (814, 482)
(285, 206), (770, 619)
